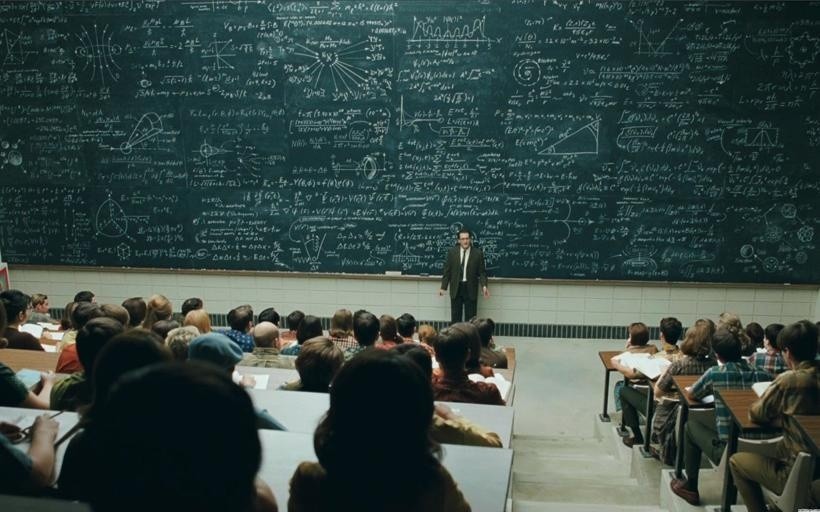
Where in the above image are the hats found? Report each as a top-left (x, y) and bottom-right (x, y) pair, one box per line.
(189, 332), (243, 371)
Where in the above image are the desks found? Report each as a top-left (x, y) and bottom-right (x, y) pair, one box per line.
(238, 388), (515, 448)
(634, 369), (676, 458)
(676, 405), (717, 469)
(234, 366), (515, 407)
(716, 388), (770, 510)
(669, 374), (718, 484)
(792, 414), (820, 452)
(616, 371), (642, 437)
(252, 430), (515, 512)
(599, 350), (629, 422)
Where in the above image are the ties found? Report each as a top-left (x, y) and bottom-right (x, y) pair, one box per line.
(460, 247), (468, 274)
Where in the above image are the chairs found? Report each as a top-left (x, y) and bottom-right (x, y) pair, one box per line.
(705, 436), (786, 504)
(761, 453), (820, 512)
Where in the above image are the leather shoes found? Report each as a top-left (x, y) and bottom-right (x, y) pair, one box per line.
(671, 478), (700, 505)
(622, 437), (643, 449)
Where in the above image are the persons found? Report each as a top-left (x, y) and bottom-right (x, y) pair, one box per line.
(0, 289), (52, 351)
(0, 338), (56, 410)
(0, 413), (60, 512)
(439, 228), (489, 324)
